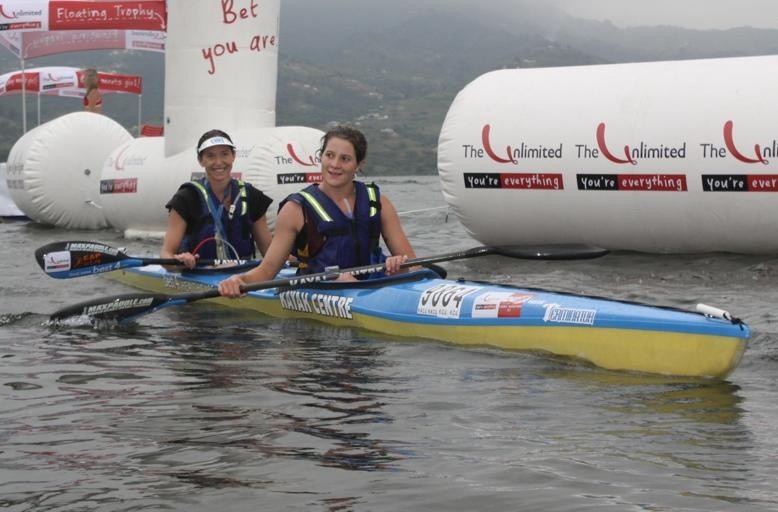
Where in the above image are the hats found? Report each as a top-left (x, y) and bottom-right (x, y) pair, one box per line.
(196, 137), (237, 153)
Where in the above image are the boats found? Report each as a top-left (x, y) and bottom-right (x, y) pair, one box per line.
(97, 244), (750, 386)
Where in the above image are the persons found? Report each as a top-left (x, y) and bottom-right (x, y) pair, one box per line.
(159, 128), (298, 275)
(215, 124), (427, 299)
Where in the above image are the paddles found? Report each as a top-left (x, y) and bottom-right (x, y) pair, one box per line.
(36, 239), (445, 278)
(49, 245), (606, 324)
